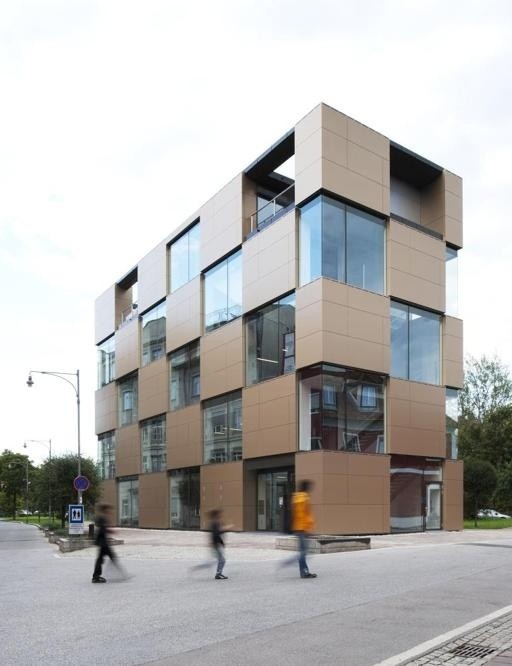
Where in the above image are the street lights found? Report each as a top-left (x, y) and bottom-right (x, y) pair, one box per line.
(1, 370), (83, 525)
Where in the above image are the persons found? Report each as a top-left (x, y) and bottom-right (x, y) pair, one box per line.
(279, 479), (319, 579)
(193, 509), (234, 580)
(91, 502), (133, 583)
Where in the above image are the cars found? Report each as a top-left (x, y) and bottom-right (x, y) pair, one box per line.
(471, 509), (512, 520)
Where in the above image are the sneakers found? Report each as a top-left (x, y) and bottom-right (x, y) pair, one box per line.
(92, 577), (106, 583)
(301, 574), (317, 578)
(215, 574), (228, 579)
